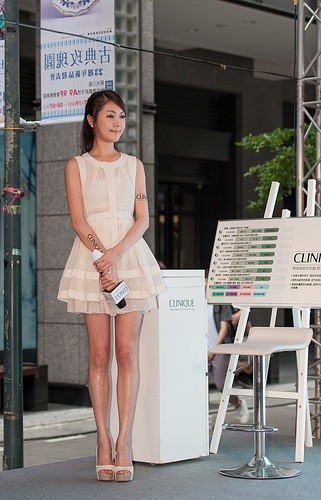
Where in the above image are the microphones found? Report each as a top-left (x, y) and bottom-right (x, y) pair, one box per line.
(100, 271), (130, 309)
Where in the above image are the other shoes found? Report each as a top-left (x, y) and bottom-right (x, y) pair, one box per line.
(238, 398), (249, 426)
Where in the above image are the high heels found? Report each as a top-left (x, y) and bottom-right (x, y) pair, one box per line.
(115, 447), (135, 481)
(95, 446), (115, 481)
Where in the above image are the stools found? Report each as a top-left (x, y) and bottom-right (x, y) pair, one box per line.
(208, 327), (314, 480)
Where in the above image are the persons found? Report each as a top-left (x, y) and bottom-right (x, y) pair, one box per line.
(56, 90), (169, 482)
(231, 304), (254, 388)
(208, 270), (250, 423)
